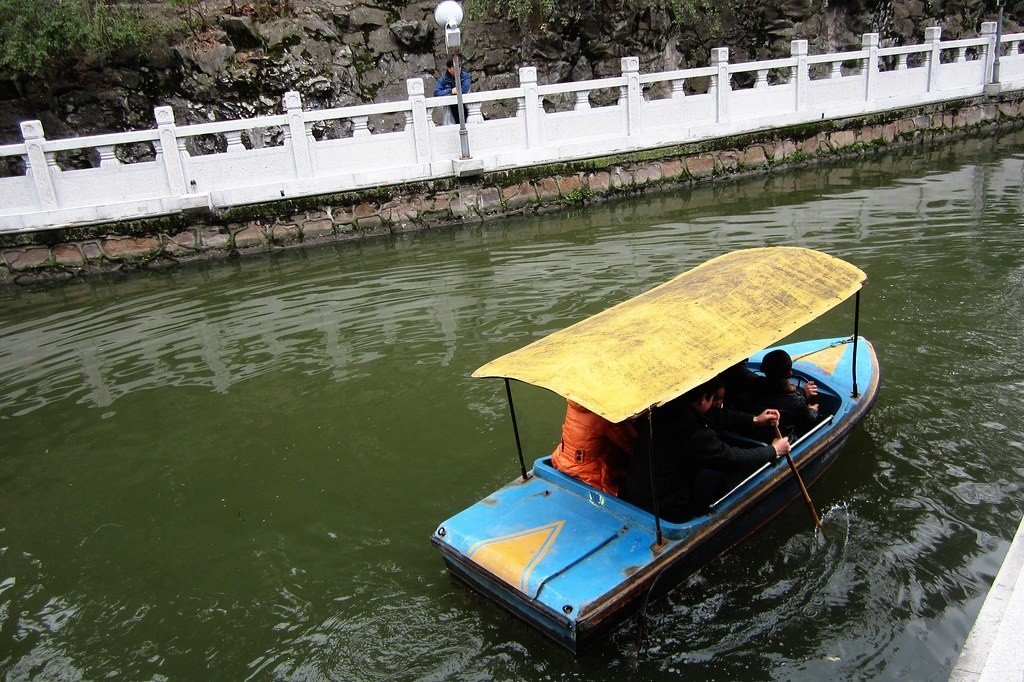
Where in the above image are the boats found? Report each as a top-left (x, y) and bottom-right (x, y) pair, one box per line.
(428, 244), (882, 661)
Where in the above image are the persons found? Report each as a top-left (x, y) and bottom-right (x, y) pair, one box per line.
(433, 58), (471, 123)
(547, 349), (825, 522)
